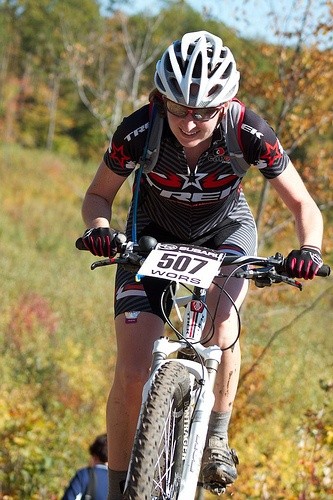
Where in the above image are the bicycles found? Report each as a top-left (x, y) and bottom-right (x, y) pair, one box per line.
(75, 232), (330, 500)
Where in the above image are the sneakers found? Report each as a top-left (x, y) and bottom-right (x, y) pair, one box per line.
(198, 429), (240, 488)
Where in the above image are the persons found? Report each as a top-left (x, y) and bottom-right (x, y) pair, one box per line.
(61, 434), (108, 500)
(80, 31), (324, 500)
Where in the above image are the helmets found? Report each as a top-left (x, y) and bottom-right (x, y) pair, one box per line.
(152, 30), (239, 108)
(88, 433), (108, 460)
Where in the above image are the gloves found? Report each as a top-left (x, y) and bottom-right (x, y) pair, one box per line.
(283, 248), (324, 281)
(82, 227), (126, 258)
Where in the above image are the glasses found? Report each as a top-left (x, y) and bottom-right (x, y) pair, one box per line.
(161, 94), (225, 121)
